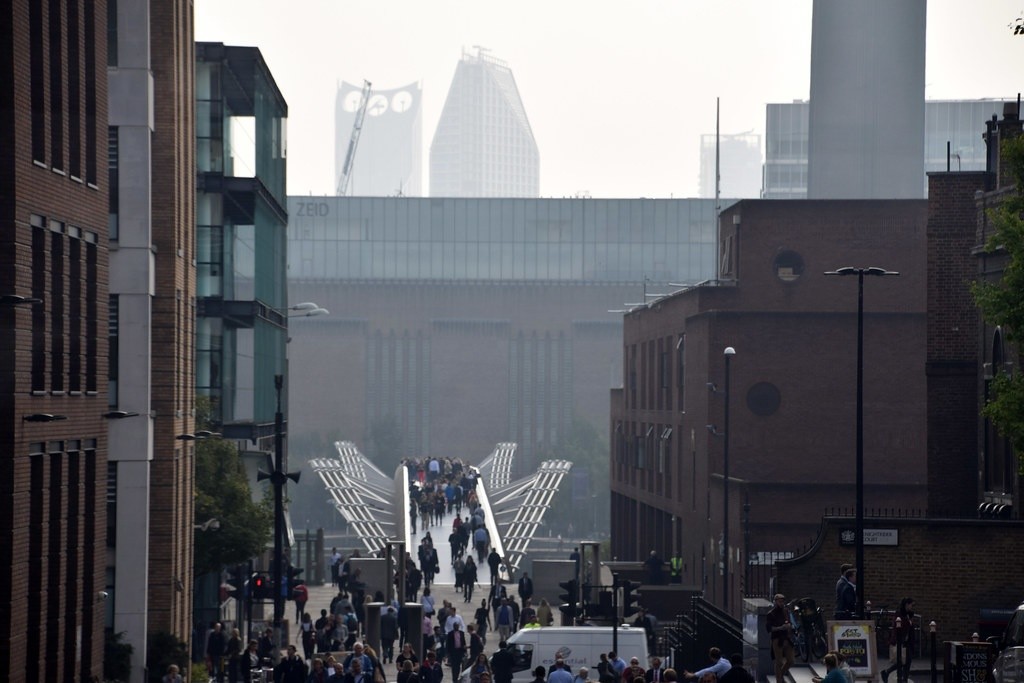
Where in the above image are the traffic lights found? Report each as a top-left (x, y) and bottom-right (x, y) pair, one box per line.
(623, 580), (641, 618)
(559, 579), (576, 615)
(288, 566), (305, 600)
(252, 575), (266, 599)
(226, 577), (244, 601)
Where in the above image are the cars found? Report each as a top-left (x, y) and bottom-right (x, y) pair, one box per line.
(986, 604), (1024, 683)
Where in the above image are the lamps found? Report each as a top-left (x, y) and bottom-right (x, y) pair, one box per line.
(195, 429), (222, 439)
(177, 432), (205, 442)
(24, 413), (66, 423)
(266, 301), (318, 312)
(281, 308), (330, 318)
(102, 410), (140, 419)
(1, 295), (44, 305)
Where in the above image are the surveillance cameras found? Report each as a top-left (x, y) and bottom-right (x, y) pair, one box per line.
(723, 347), (736, 360)
(210, 521), (219, 530)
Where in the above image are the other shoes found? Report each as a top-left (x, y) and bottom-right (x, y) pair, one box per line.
(881, 669), (889, 683)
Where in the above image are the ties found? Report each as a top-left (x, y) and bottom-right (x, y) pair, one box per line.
(655, 670), (658, 681)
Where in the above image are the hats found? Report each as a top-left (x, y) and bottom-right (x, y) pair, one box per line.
(773, 594), (784, 599)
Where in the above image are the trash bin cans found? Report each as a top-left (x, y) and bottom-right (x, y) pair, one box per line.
(268, 619), (290, 649)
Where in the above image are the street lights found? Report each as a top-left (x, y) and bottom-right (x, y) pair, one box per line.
(722, 346), (736, 613)
(822, 267), (900, 622)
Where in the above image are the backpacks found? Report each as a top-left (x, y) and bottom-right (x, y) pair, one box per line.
(345, 614), (357, 632)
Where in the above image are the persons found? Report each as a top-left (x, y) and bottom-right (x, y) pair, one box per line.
(156, 457), (923, 683)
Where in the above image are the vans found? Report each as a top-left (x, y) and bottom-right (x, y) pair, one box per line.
(457, 626), (649, 683)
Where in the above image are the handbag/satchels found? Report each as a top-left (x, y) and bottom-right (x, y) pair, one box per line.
(546, 605), (553, 623)
(434, 564), (440, 573)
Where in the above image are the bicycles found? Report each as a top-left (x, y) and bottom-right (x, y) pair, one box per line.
(785, 598), (828, 662)
